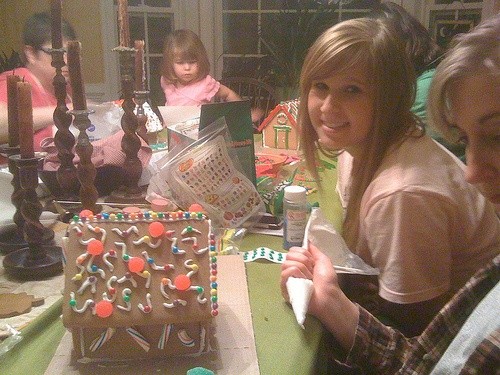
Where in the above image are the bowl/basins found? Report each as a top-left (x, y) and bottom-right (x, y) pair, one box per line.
(37, 157), (104, 194)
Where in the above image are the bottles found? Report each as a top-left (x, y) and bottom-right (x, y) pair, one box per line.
(282, 185), (306, 249)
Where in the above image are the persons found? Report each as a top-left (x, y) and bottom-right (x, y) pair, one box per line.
(0, 11), (99, 174)
(159, 27), (245, 112)
(281, 0), (500, 374)
